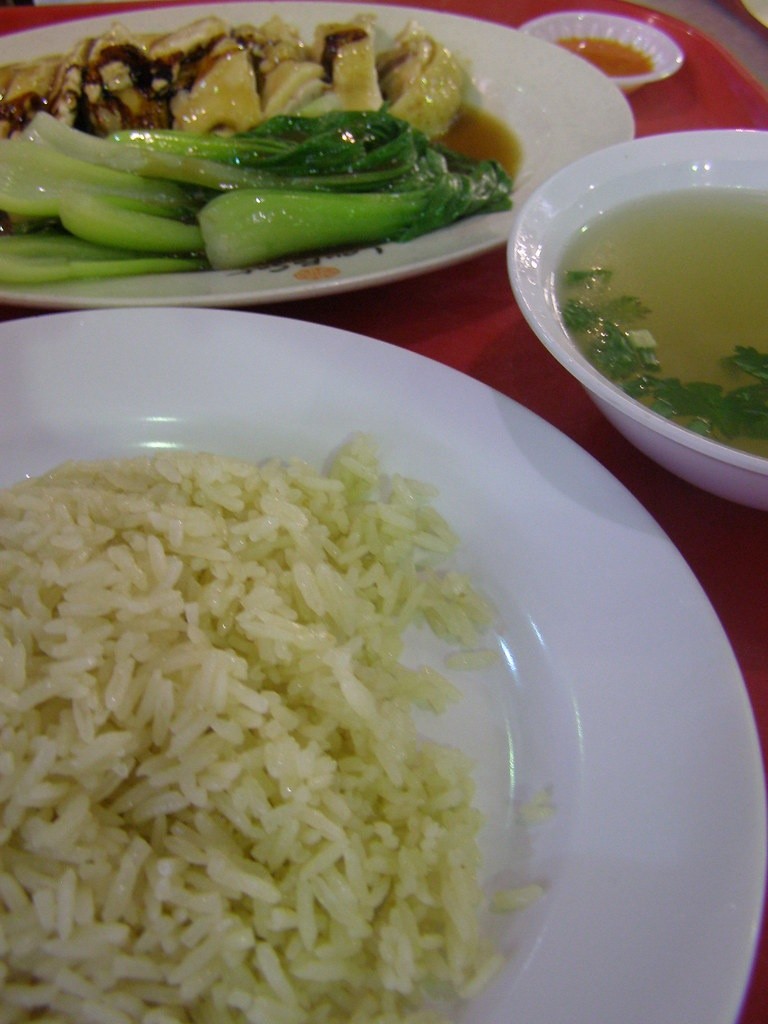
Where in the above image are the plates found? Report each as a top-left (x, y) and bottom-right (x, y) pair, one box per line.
(0, 308), (768, 1024)
(741, 0), (768, 29)
(0, 0), (635, 296)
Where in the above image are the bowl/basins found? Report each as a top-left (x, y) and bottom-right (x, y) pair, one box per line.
(519, 12), (685, 95)
(507, 125), (768, 514)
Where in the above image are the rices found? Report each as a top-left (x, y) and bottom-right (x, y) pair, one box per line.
(0, 428), (559, 1024)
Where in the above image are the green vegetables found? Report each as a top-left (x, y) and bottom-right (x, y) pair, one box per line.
(0, 101), (515, 285)
(560, 266), (767, 447)
